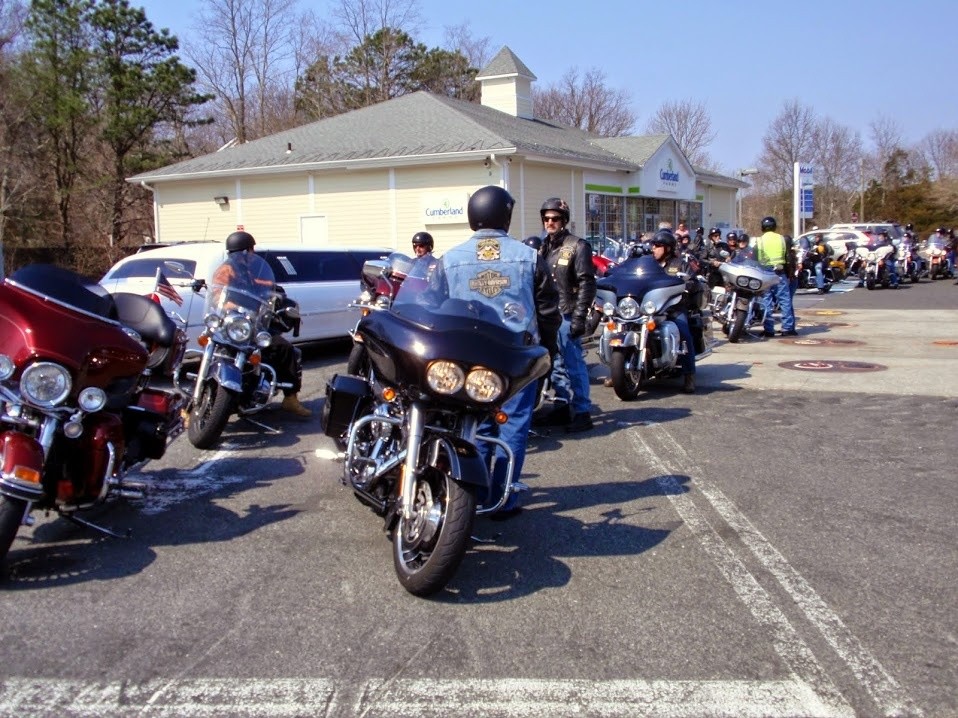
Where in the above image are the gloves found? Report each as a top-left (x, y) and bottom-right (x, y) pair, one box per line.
(570, 316), (585, 338)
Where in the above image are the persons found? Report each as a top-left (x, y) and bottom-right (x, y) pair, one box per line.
(757, 212), (798, 337)
(652, 228), (702, 394)
(674, 224), (754, 274)
(811, 233), (833, 294)
(214, 232), (313, 418)
(538, 197), (596, 433)
(425, 186), (563, 520)
(412, 232), (448, 298)
(878, 224), (958, 284)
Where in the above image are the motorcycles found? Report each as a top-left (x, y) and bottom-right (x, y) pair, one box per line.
(855, 233), (953, 290)
(322, 252), (569, 600)
(163, 251), (297, 450)
(791, 246), (847, 294)
(1, 263), (193, 568)
(711, 247), (781, 344)
(591, 235), (720, 402)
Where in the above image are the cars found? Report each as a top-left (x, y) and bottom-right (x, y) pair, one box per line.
(789, 228), (875, 264)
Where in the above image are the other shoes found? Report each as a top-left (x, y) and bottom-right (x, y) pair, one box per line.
(782, 330), (798, 335)
(855, 283), (863, 287)
(491, 506), (522, 522)
(894, 283), (899, 288)
(818, 288), (826, 295)
(565, 412), (593, 431)
(535, 404), (571, 426)
(760, 331), (774, 337)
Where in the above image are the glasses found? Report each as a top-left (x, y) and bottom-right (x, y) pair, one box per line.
(543, 216), (562, 222)
(413, 243), (425, 248)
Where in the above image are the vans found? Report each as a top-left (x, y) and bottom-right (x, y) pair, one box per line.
(96, 241), (395, 360)
(833, 222), (905, 249)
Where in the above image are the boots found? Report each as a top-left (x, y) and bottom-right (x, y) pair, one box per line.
(684, 375), (694, 393)
(604, 377), (613, 387)
(283, 394), (312, 418)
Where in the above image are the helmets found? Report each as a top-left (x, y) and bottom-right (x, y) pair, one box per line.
(412, 232), (433, 248)
(761, 217), (776, 231)
(468, 186), (516, 230)
(650, 228), (748, 251)
(815, 233), (823, 242)
(936, 227), (954, 234)
(525, 236), (541, 250)
(540, 197), (569, 225)
(906, 223), (913, 230)
(904, 233), (911, 239)
(879, 229), (887, 235)
(226, 231), (256, 250)
